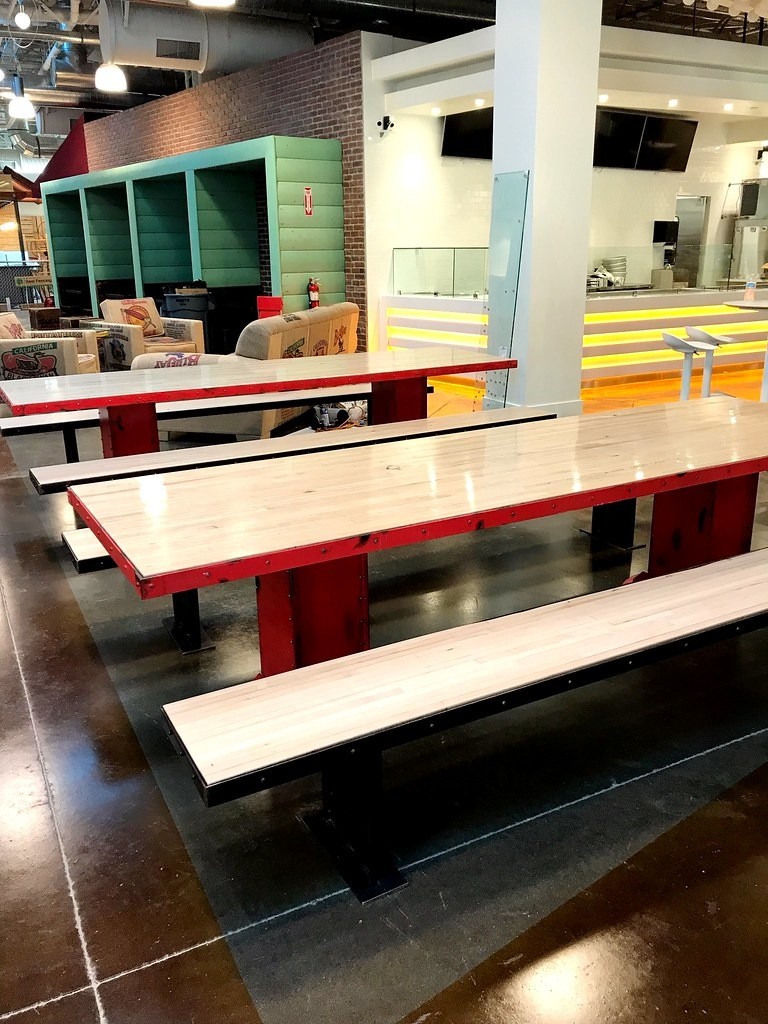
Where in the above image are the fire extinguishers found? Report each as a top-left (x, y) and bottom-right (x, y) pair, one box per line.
(307, 276), (323, 307)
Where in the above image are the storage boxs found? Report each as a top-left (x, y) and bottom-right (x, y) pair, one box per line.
(176, 288), (207, 294)
(59, 316), (87, 328)
(29, 306), (61, 330)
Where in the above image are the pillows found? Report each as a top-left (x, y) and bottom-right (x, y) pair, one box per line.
(99, 296), (164, 337)
(0, 311), (29, 338)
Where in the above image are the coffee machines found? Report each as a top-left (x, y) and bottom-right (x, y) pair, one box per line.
(650, 216), (688, 288)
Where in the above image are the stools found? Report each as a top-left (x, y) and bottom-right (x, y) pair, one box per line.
(660, 332), (715, 400)
(684, 326), (737, 399)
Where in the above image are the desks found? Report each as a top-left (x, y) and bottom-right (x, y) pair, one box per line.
(722, 301), (767, 405)
(1, 344), (521, 460)
(68, 393), (768, 678)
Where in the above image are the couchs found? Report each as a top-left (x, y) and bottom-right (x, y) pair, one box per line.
(129, 297), (361, 444)
(78, 317), (204, 372)
(0, 330), (102, 404)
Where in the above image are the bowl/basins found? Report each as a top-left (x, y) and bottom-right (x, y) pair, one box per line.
(602, 256), (627, 286)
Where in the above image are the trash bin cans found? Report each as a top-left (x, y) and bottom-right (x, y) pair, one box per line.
(164, 292), (209, 353)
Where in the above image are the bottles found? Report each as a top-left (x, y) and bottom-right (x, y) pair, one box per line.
(744, 274), (756, 303)
(319, 405), (329, 427)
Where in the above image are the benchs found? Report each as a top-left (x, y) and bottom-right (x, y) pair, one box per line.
(161, 543), (767, 906)
(0, 377), (433, 496)
(30, 402), (558, 495)
(59, 498), (636, 655)
